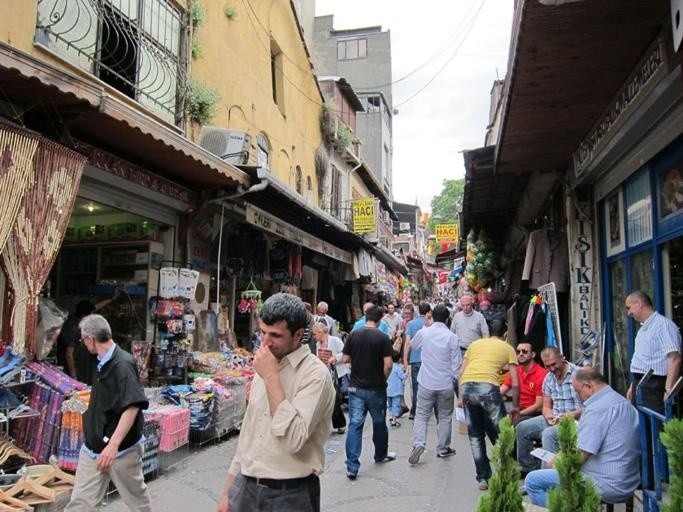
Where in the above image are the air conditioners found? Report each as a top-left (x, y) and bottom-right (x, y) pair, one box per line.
(400, 222), (410, 231)
(196, 125), (254, 165)
(188, 273), (211, 313)
(383, 210), (390, 225)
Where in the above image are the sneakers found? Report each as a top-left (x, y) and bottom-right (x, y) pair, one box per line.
(437, 447), (456, 457)
(478, 479), (487, 490)
(396, 405), (409, 415)
(391, 422), (400, 429)
(376, 452), (396, 463)
(407, 446), (424, 464)
(337, 426), (346, 434)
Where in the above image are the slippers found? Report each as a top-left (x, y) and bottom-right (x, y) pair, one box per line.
(223, 346), (254, 375)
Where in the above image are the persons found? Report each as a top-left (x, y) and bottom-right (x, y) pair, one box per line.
(62, 298), (106, 384)
(523, 365), (641, 509)
(498, 337), (549, 461)
(515, 346), (586, 468)
(62, 314), (151, 512)
(298, 278), (510, 479)
(218, 294), (335, 512)
(456, 318), (520, 491)
(624, 289), (683, 490)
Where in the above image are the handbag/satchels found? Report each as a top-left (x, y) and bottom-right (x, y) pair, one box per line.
(327, 363), (338, 394)
(392, 336), (402, 353)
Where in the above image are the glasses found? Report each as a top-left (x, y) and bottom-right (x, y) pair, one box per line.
(79, 336), (89, 345)
(517, 349), (527, 354)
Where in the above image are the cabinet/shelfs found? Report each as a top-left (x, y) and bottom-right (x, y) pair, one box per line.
(48, 239), (164, 386)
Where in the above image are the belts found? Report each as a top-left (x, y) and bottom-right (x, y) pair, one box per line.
(246, 473), (312, 490)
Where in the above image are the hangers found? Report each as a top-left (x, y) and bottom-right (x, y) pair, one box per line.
(1, 339), (77, 511)
